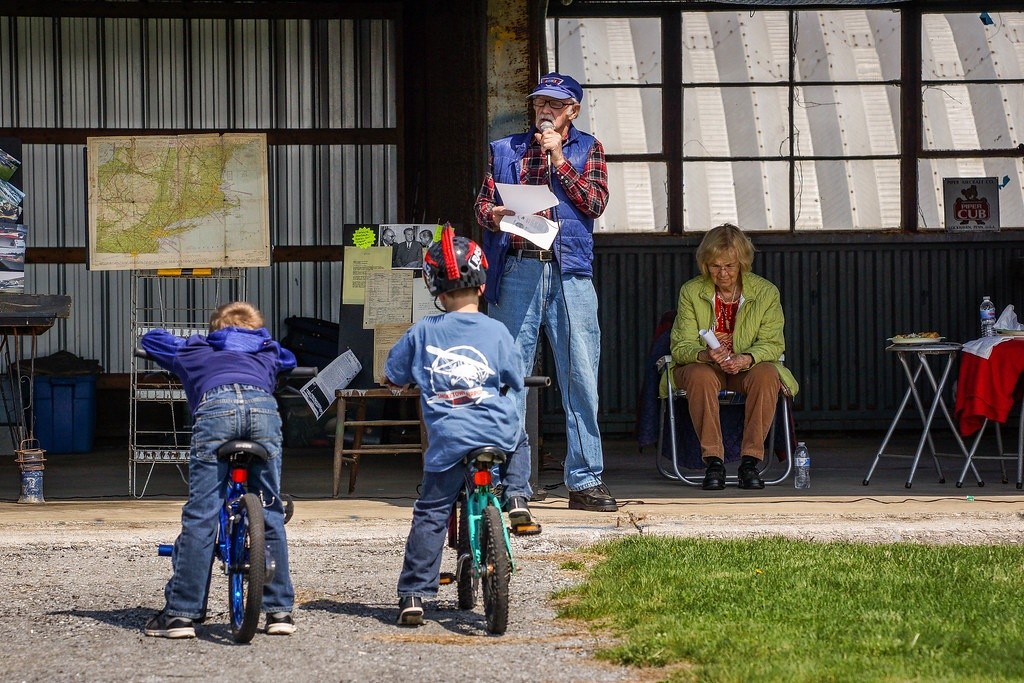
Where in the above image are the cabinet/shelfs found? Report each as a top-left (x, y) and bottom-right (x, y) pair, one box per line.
(127, 269), (246, 498)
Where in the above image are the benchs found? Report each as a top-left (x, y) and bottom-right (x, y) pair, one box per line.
(94, 372), (187, 392)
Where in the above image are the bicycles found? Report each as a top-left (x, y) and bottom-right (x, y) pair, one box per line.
(132, 347), (317, 643)
(380, 375), (551, 634)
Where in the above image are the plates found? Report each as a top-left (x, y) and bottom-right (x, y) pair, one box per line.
(886, 337), (946, 343)
(997, 329), (1024, 336)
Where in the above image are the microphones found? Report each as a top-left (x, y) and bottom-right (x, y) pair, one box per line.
(540, 121), (553, 165)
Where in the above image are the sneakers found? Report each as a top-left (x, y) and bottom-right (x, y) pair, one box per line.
(264, 611), (297, 633)
(569, 486), (617, 511)
(703, 460), (726, 489)
(508, 497), (537, 528)
(738, 465), (764, 488)
(144, 609), (195, 637)
(396, 596), (423, 624)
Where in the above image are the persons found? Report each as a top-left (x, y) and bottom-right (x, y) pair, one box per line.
(395, 228), (423, 267)
(473, 72), (618, 512)
(380, 228), (399, 267)
(383, 223), (544, 626)
(660, 223), (799, 490)
(141, 300), (297, 638)
(418, 229), (438, 259)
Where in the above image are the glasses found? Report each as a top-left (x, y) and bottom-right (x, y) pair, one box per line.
(532, 98), (574, 109)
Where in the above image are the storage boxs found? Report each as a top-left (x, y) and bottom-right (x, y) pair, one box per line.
(22, 374), (96, 453)
(278, 394), (336, 449)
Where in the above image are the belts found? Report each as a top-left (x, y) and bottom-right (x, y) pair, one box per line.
(507, 249), (556, 261)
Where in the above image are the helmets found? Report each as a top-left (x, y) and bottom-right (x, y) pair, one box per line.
(423, 236), (488, 296)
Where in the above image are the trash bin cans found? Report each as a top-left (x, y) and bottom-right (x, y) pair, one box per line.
(17, 350), (104, 454)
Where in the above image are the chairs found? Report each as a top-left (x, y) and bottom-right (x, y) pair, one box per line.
(639, 310), (796, 489)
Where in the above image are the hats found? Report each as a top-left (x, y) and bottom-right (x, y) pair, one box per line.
(526, 72), (583, 103)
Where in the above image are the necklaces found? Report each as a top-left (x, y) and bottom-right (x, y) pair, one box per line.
(716, 275), (742, 330)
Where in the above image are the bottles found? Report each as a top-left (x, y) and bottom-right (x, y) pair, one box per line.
(794, 442), (810, 489)
(980, 296), (996, 338)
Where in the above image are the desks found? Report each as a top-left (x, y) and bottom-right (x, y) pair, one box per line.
(332, 388), (428, 496)
(0, 292), (76, 494)
(952, 331), (1024, 490)
(862, 341), (985, 487)
